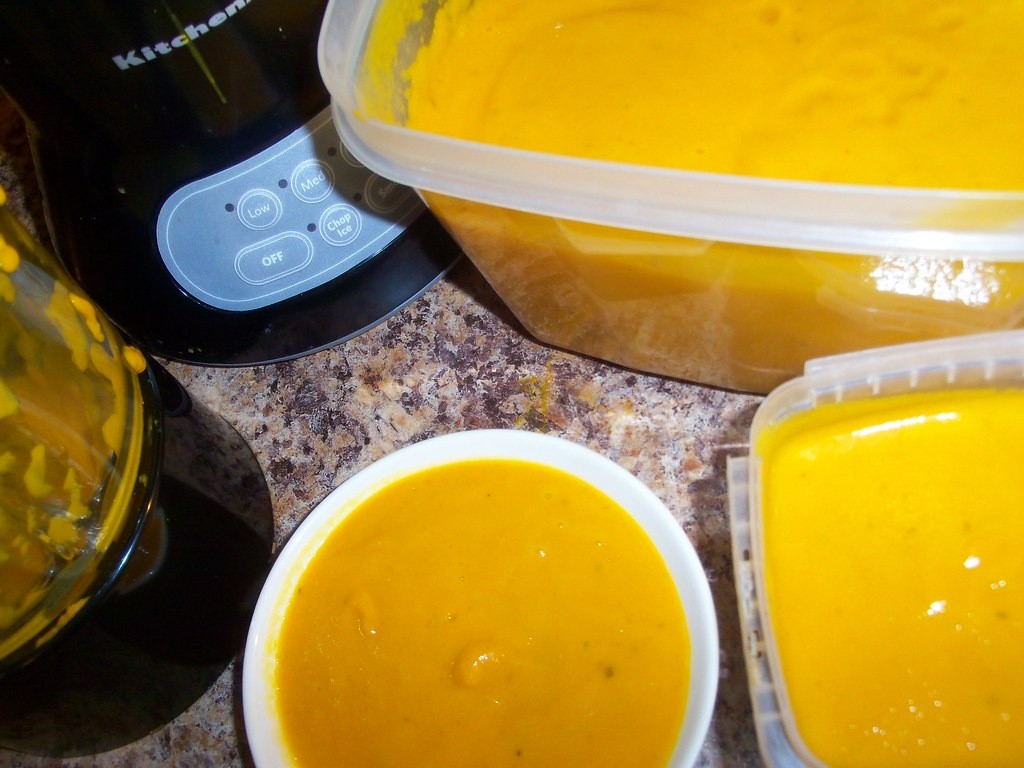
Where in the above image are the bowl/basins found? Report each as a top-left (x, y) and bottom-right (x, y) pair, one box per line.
(237, 429), (721, 768)
(725, 327), (1024, 768)
(311, 0), (1023, 395)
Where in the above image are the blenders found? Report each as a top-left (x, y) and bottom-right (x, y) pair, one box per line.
(0, 0), (466, 761)
